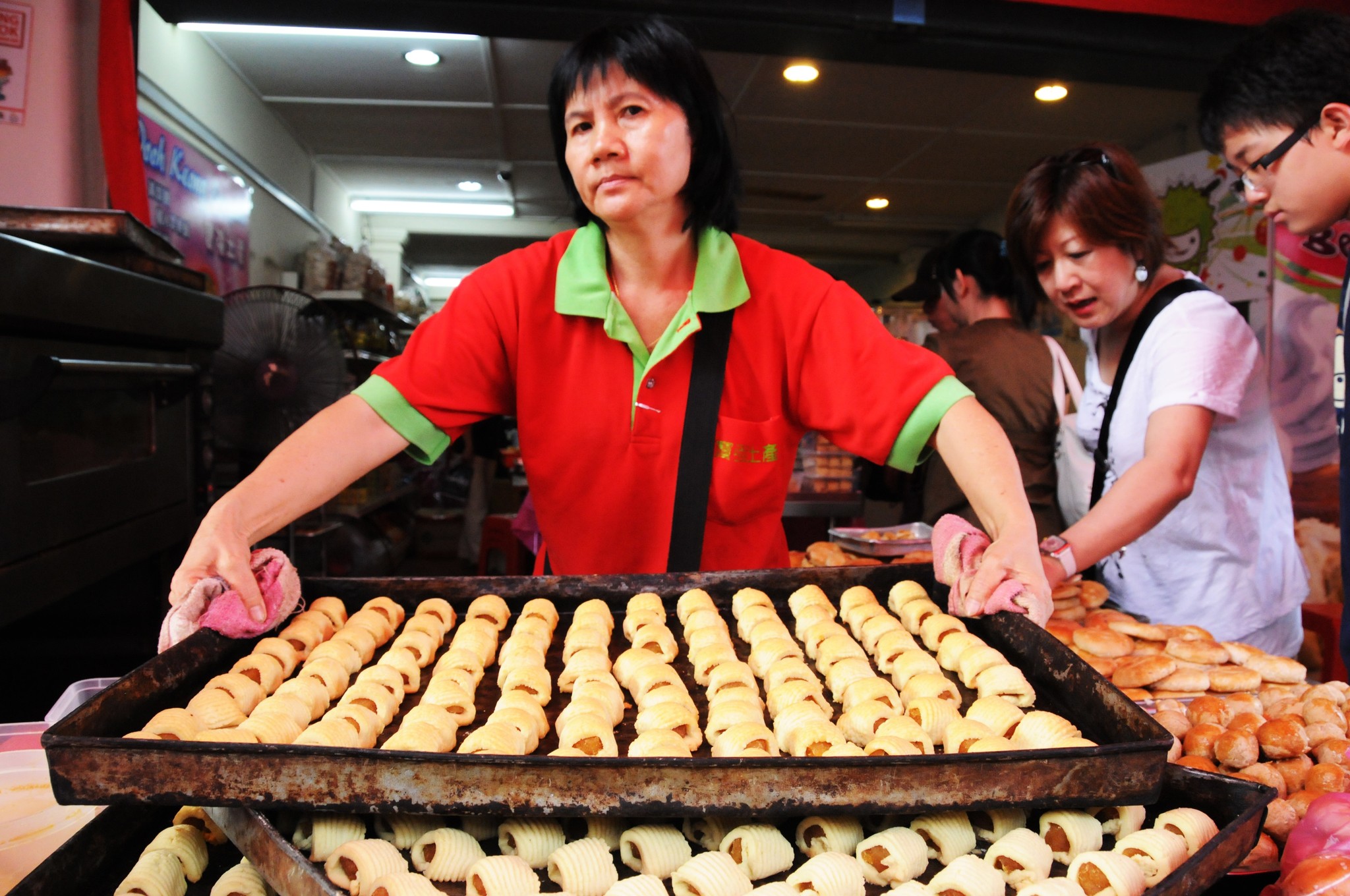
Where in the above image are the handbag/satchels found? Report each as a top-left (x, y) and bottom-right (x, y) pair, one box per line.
(1039, 332), (1095, 527)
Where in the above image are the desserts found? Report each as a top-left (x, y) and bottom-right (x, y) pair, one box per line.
(111, 582), (1220, 895)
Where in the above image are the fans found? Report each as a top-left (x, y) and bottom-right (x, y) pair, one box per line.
(200, 285), (365, 473)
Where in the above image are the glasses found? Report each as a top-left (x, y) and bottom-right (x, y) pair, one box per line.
(1229, 110), (1324, 205)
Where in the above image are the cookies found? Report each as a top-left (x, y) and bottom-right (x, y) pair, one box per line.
(1042, 574), (1311, 700)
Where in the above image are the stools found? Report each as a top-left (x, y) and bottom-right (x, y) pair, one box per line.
(474, 512), (537, 575)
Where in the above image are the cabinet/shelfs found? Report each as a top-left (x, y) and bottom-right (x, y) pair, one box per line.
(310, 289), (417, 362)
(288, 444), (447, 575)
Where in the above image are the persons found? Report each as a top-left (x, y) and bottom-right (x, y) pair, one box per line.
(999, 133), (1306, 662)
(1193, 31), (1350, 689)
(888, 223), (1089, 553)
(163, 12), (1061, 632)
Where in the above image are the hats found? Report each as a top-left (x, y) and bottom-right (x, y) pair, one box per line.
(891, 249), (945, 304)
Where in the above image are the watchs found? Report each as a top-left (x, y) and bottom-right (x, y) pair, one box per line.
(1036, 530), (1078, 582)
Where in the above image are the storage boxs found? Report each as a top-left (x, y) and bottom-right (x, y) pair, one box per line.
(0, 676), (126, 895)
(415, 507), (467, 557)
(329, 459), (404, 504)
